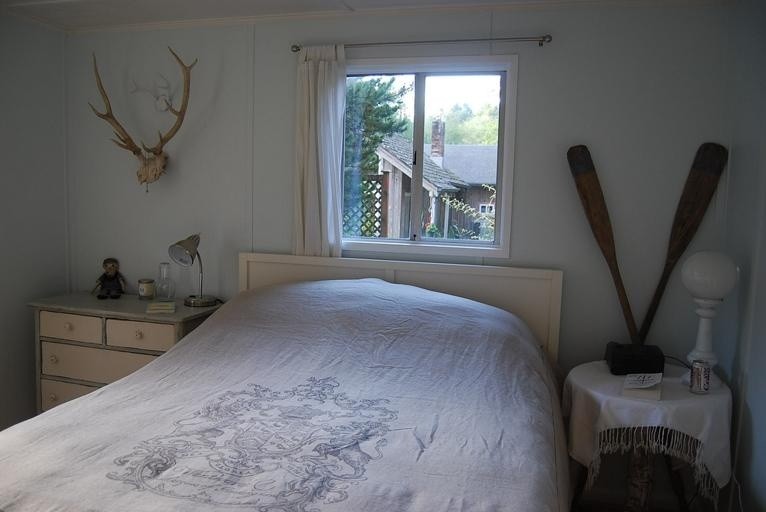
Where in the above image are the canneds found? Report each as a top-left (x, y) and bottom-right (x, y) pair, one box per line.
(689, 359), (712, 394)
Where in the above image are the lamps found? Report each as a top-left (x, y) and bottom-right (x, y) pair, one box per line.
(681, 251), (737, 389)
(169, 234), (216, 307)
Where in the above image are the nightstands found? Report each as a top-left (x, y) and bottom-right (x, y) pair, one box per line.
(27, 291), (221, 414)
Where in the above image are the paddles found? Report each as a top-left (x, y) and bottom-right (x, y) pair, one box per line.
(637, 142), (729, 345)
(565, 144), (638, 345)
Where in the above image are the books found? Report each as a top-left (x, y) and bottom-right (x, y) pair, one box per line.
(622, 372), (664, 401)
(146, 301), (176, 315)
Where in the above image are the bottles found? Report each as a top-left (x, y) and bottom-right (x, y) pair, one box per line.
(156, 261), (177, 303)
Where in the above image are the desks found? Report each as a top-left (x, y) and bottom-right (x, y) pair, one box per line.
(561, 361), (732, 511)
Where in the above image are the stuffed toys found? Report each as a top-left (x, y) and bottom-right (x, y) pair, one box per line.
(89, 257), (127, 300)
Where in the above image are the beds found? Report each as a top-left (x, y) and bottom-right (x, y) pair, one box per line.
(0, 252), (570, 512)
